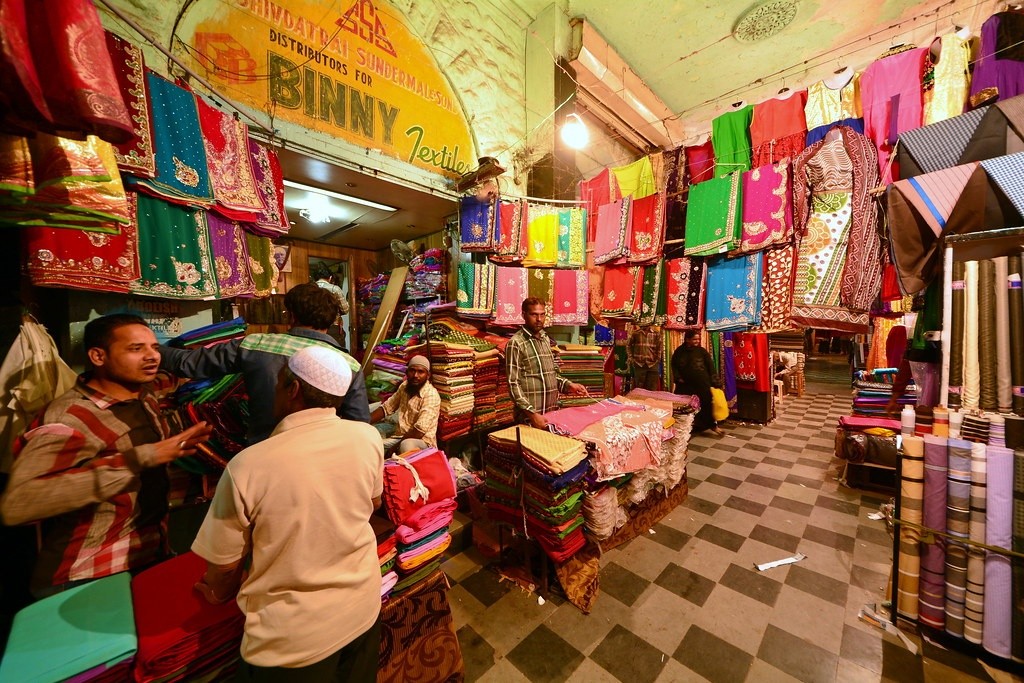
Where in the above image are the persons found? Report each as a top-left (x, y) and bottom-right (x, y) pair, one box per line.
(316, 268), (350, 350)
(505, 297), (589, 429)
(773, 351), (798, 395)
(671, 329), (725, 437)
(626, 325), (662, 392)
(273, 246), (287, 270)
(191, 345), (384, 683)
(156, 282), (370, 444)
(369, 355), (441, 460)
(1, 313), (213, 593)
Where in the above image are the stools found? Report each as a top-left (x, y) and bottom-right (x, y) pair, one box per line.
(790, 371), (804, 399)
(774, 380), (784, 405)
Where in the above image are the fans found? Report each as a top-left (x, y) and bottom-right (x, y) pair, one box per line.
(390, 239), (420, 264)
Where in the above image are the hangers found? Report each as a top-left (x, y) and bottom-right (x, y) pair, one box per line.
(833, 55), (848, 75)
(950, 10), (963, 33)
(776, 77), (790, 95)
(726, 138), (798, 176)
(686, 157), (746, 187)
(596, 176), (668, 207)
(887, 35), (905, 51)
(731, 93), (742, 108)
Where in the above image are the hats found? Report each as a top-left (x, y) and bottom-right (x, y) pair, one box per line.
(287, 346), (352, 397)
(408, 354), (431, 371)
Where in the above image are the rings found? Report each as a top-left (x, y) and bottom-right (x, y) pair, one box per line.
(177, 441), (187, 449)
